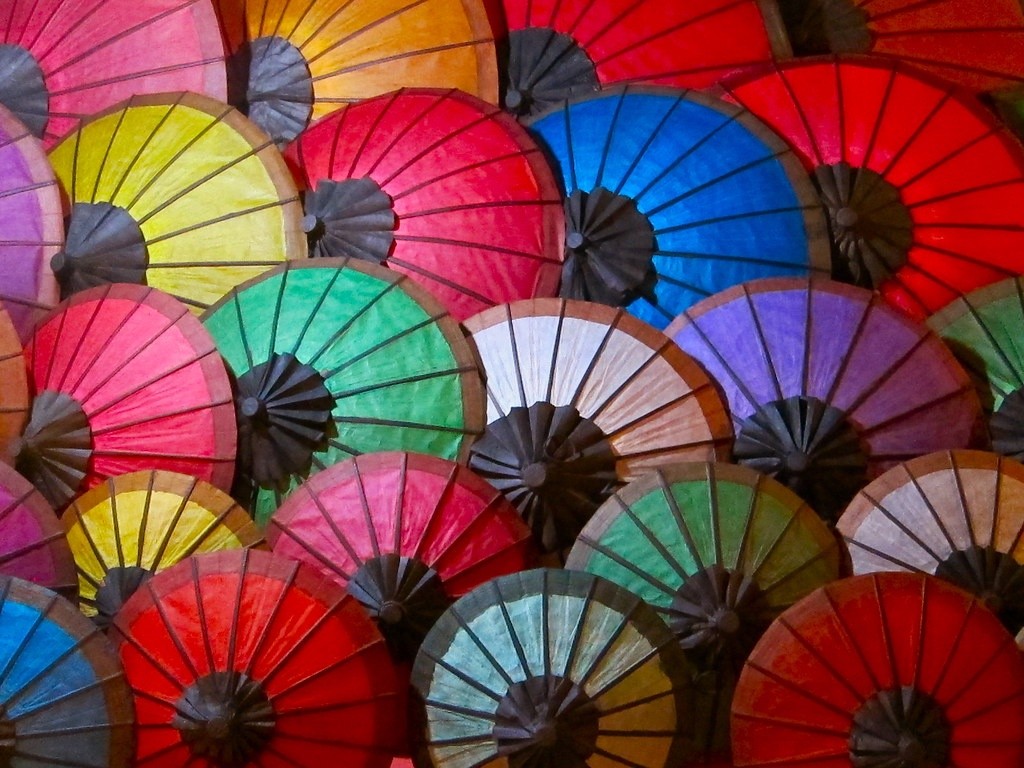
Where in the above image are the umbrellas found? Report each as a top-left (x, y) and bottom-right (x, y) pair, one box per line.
(0, 0), (1024, 768)
